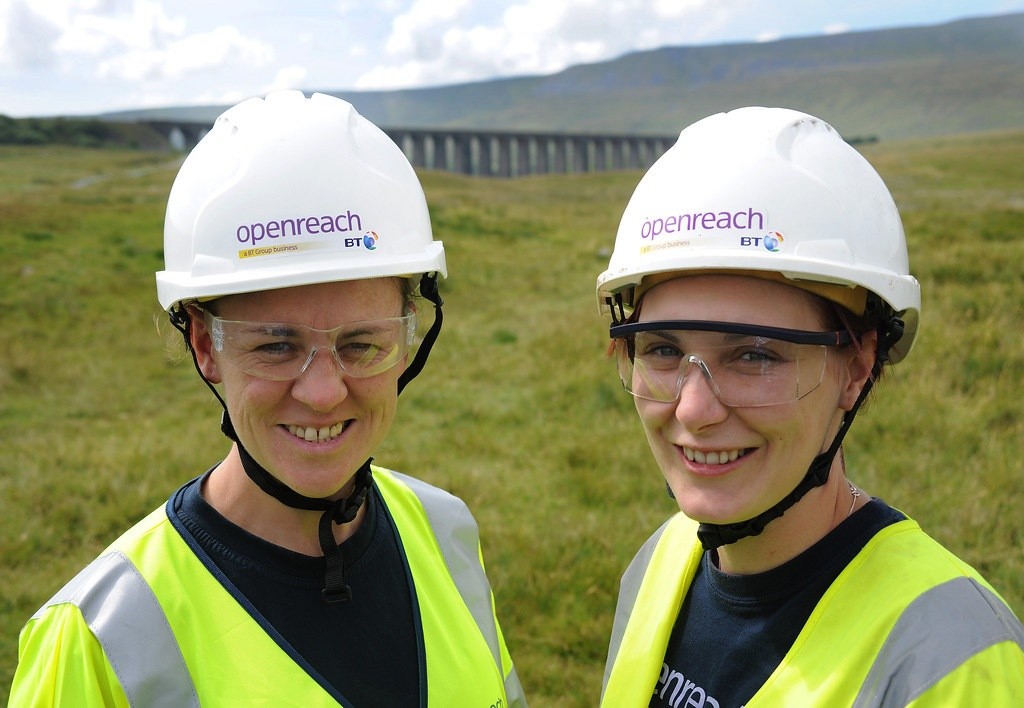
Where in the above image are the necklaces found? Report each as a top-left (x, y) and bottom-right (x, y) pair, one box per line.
(848, 483), (861, 515)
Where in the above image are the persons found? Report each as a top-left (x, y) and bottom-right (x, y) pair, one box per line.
(6, 89), (528, 708)
(594, 107), (1024, 708)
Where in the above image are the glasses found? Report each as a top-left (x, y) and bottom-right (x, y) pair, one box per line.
(614, 319), (829, 407)
(185, 300), (418, 382)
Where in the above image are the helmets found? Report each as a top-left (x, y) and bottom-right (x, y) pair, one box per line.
(154, 90), (449, 310)
(597, 105), (921, 362)
